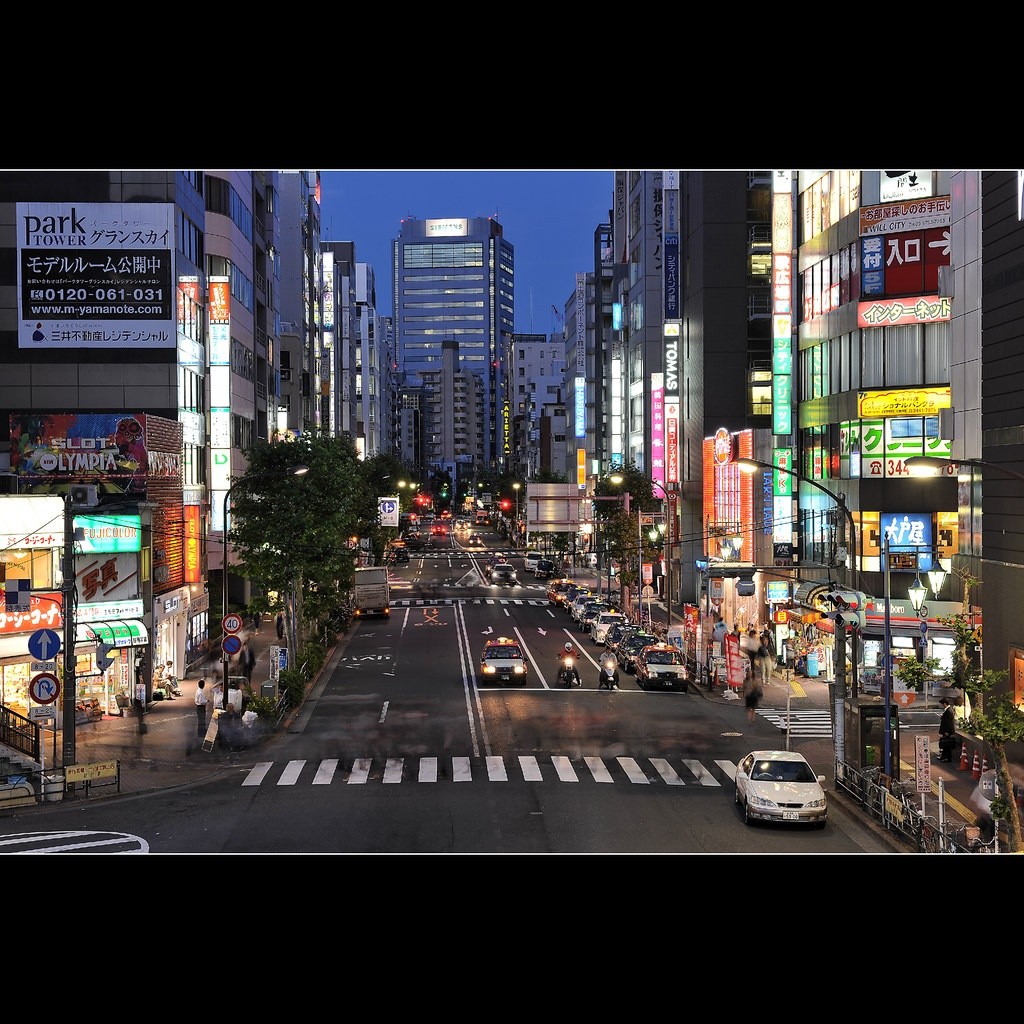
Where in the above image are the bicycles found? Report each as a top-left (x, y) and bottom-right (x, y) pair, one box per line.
(861, 762), (999, 853)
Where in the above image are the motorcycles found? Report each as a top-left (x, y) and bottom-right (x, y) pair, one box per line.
(554, 652), (582, 689)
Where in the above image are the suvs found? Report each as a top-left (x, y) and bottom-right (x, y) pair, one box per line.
(392, 546), (410, 562)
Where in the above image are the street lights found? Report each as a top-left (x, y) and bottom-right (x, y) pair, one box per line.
(512, 482), (520, 530)
(223, 465), (310, 711)
(610, 474), (673, 630)
(737, 457), (893, 790)
(648, 520), (667, 602)
(879, 530), (948, 777)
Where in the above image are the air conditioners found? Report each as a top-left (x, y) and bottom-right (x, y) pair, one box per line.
(69, 483), (99, 508)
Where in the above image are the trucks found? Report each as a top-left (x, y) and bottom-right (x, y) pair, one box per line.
(348, 565), (392, 621)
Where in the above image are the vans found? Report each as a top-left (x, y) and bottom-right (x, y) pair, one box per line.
(523, 551), (547, 573)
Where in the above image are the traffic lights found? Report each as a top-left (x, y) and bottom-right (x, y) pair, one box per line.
(399, 482), (416, 490)
(820, 610), (859, 629)
(419, 498), (430, 502)
(441, 485), (448, 498)
(502, 500), (508, 510)
(818, 590), (859, 610)
(103, 643), (116, 658)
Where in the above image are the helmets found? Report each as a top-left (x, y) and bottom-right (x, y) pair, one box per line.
(605, 646), (612, 650)
(565, 642), (573, 652)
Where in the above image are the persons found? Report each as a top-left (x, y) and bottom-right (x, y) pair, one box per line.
(238, 643), (256, 684)
(742, 670), (759, 722)
(195, 679), (210, 737)
(598, 646), (621, 689)
(252, 611), (264, 635)
(154, 661), (183, 701)
(698, 593), (776, 685)
(936, 696), (964, 763)
(221, 704), (242, 752)
(274, 612), (286, 640)
(557, 643), (580, 685)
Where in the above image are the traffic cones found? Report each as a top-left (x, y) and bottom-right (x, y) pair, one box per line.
(956, 741), (971, 772)
(968, 748), (982, 781)
(981, 754), (989, 775)
(1015, 783), (1024, 807)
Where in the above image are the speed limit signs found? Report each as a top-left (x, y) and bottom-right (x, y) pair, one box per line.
(222, 613), (243, 635)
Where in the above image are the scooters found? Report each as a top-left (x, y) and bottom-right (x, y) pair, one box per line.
(598, 657), (622, 690)
(389, 556), (398, 567)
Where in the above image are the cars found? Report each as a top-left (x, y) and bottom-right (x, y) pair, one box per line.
(635, 645), (691, 694)
(534, 560), (555, 579)
(480, 638), (529, 686)
(484, 558), (508, 577)
(546, 578), (645, 654)
(613, 633), (662, 674)
(398, 498), (533, 558)
(490, 564), (517, 585)
(735, 750), (828, 829)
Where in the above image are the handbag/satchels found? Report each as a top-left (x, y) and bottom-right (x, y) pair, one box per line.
(242, 712), (258, 728)
(770, 659), (777, 670)
(152, 692), (164, 701)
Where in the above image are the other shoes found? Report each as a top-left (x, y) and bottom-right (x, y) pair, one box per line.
(615, 683), (619, 688)
(941, 758), (951, 762)
(577, 682), (580, 685)
(937, 756), (942, 759)
(599, 683), (603, 687)
(168, 697), (176, 700)
